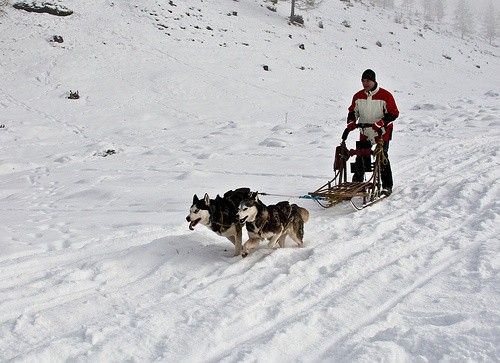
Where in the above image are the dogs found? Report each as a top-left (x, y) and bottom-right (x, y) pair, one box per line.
(185, 187), (309, 258)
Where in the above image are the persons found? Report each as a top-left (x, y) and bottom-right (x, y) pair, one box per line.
(347, 68), (400, 197)
